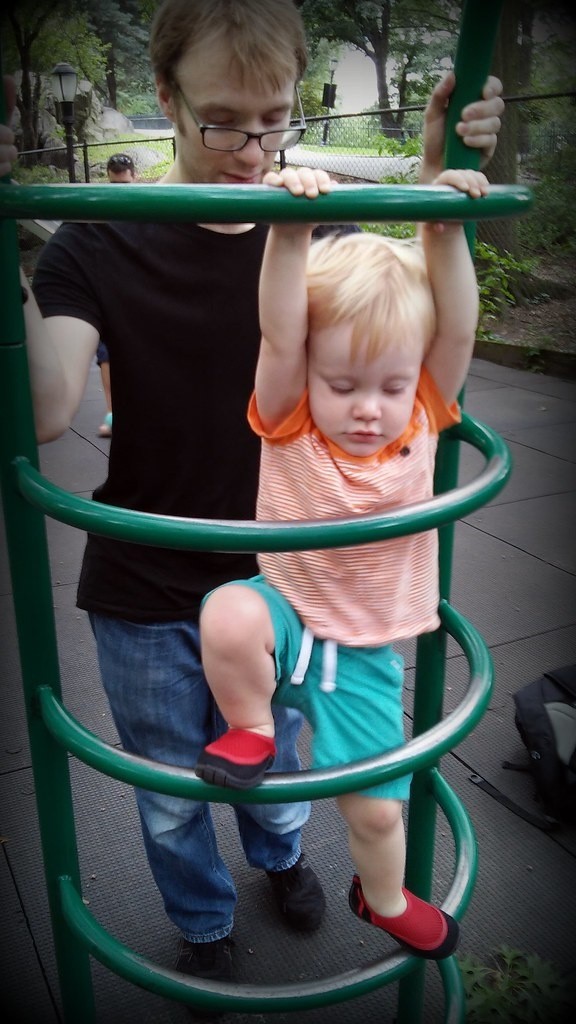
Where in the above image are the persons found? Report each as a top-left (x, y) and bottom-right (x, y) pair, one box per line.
(200, 167), (490, 960)
(95, 153), (136, 437)
(0, 0), (503, 1014)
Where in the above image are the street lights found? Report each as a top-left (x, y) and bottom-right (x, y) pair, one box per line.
(322, 56), (339, 144)
(49, 62), (77, 182)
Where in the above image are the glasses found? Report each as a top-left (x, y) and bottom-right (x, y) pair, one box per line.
(108, 156), (131, 166)
(169, 68), (308, 152)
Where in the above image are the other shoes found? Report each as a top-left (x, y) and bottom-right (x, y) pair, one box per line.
(195, 727), (278, 790)
(348, 873), (461, 960)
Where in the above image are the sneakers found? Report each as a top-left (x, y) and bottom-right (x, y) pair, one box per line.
(177, 933), (238, 1017)
(267, 851), (327, 928)
(98, 412), (112, 436)
